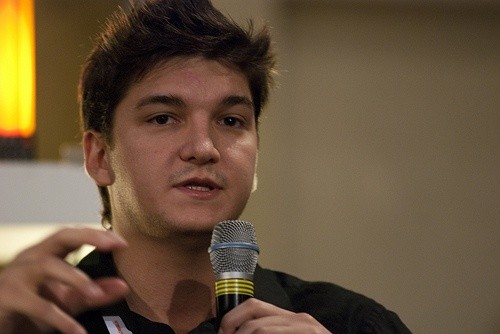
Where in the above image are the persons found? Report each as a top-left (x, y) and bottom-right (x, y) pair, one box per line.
(1, 0), (416, 334)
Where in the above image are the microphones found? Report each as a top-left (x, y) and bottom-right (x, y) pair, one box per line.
(208, 220), (259, 334)
